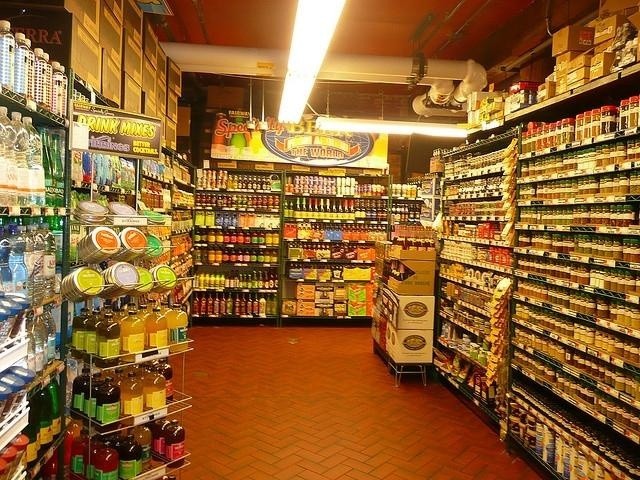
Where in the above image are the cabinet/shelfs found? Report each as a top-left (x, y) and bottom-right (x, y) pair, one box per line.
(138, 146), (195, 323)
(391, 175), (421, 221)
(62, 65), (138, 479)
(280, 171), (390, 327)
(195, 167), (283, 327)
(506, 127), (639, 480)
(64, 209), (194, 479)
(421, 174), (441, 223)
(1, 67), (73, 480)
(434, 123), (522, 430)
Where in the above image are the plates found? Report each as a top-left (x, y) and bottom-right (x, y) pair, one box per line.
(59, 201), (179, 304)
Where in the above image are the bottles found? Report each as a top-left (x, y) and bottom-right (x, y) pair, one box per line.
(73, 152), (165, 209)
(163, 148), (195, 310)
(1, 106), (66, 479)
(0, 18), (90, 118)
(195, 169), (281, 229)
(64, 302), (189, 479)
(192, 269), (279, 317)
(284, 171), (433, 281)
(433, 82), (640, 479)
(212, 113), (264, 161)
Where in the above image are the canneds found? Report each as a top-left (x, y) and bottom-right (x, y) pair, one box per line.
(511, 95), (640, 437)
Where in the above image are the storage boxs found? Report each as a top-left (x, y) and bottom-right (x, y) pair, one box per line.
(0, 0), (182, 151)
(466, 91), (505, 129)
(537, 0), (640, 103)
(374, 224), (436, 365)
